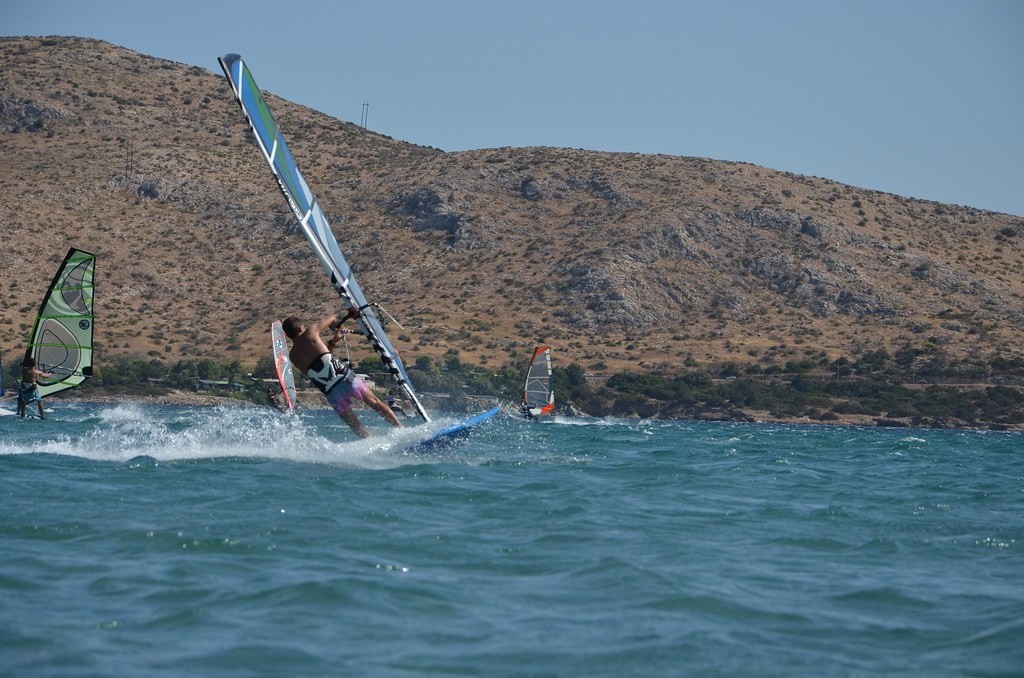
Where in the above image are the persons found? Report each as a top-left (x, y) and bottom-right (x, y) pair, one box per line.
(282, 316), (404, 440)
(267, 387), (285, 414)
(387, 390), (411, 418)
(20, 358), (55, 419)
(520, 397), (537, 420)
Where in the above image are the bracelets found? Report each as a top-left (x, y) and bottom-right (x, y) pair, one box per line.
(327, 340), (335, 346)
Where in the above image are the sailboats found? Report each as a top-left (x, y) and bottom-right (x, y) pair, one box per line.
(521, 345), (565, 424)
(217, 54), (500, 457)
(228, 320), (305, 421)
(0, 247), (97, 422)
